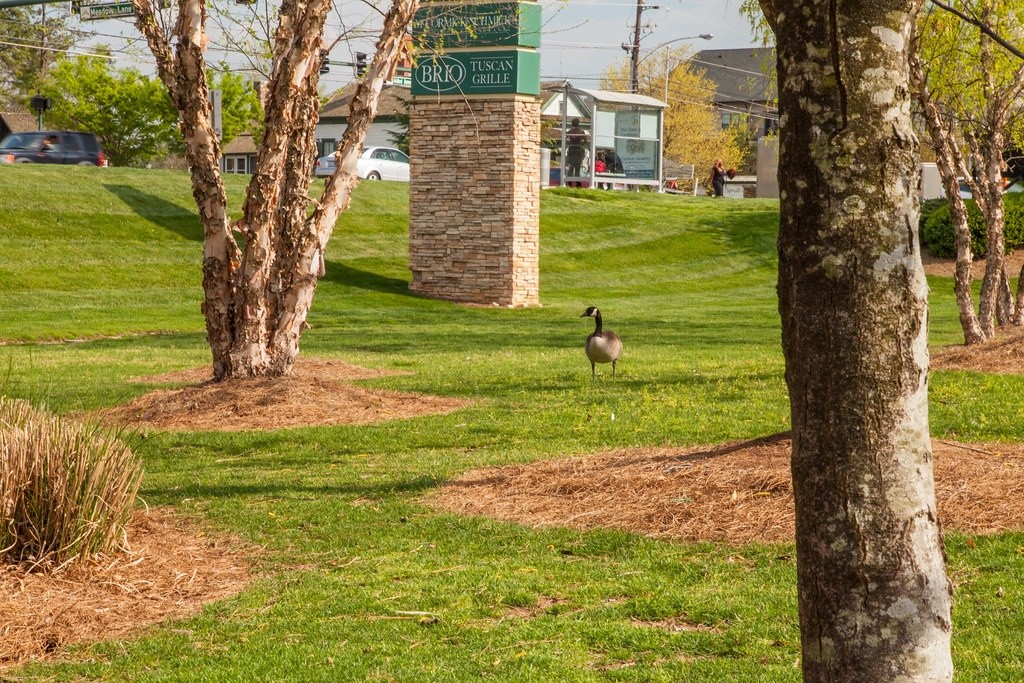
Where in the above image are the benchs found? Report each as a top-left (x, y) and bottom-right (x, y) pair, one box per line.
(584, 171), (626, 189)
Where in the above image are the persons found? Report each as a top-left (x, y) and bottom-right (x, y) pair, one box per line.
(712, 158), (724, 197)
(595, 149), (624, 190)
(43, 139), (52, 145)
(566, 118), (587, 187)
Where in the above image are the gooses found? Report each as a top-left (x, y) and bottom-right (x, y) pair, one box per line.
(580, 307), (623, 376)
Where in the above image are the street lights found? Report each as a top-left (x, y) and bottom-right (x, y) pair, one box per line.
(629, 34), (714, 94)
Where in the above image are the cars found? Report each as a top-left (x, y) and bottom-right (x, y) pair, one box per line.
(940, 177), (1024, 200)
(550, 167), (589, 189)
(315, 146), (410, 183)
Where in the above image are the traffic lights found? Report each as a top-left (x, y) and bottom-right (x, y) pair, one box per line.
(44, 98), (52, 110)
(32, 96), (44, 110)
(356, 51), (368, 77)
(318, 49), (331, 74)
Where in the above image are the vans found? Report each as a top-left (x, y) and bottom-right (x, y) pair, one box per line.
(0, 129), (105, 169)
